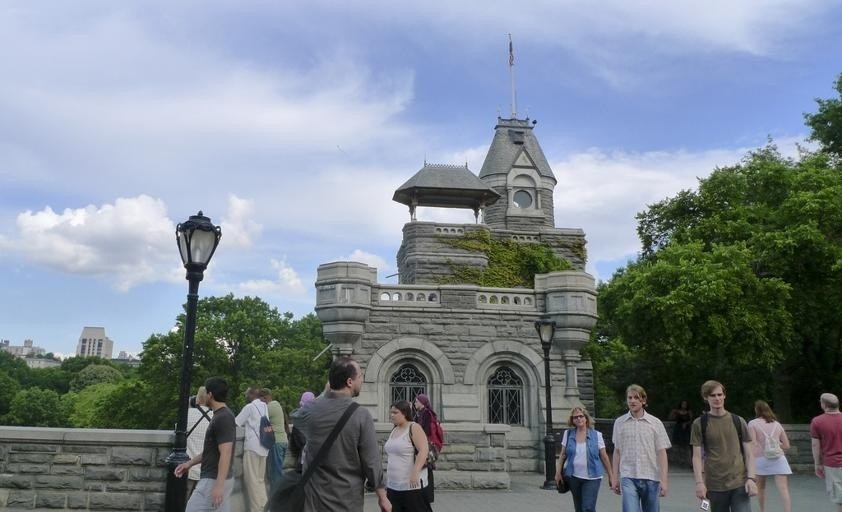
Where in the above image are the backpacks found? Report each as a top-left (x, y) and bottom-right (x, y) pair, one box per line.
(263, 463), (306, 512)
(246, 401), (276, 450)
(750, 419), (783, 459)
(556, 475), (571, 494)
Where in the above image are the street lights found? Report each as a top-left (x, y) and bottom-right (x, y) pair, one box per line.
(162, 210), (225, 510)
(532, 315), (562, 491)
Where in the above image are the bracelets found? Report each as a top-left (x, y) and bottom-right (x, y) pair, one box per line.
(814, 462), (822, 466)
(747, 475), (757, 482)
(695, 481), (704, 484)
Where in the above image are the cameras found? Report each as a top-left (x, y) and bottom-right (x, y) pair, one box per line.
(701, 499), (709, 511)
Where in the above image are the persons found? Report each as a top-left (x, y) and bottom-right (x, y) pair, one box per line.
(811, 392), (842, 512)
(747, 400), (794, 512)
(611, 384), (671, 511)
(554, 404), (612, 512)
(668, 398), (694, 465)
(172, 358), (443, 511)
(691, 379), (758, 512)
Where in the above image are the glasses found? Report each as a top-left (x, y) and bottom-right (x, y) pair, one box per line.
(572, 415), (585, 419)
